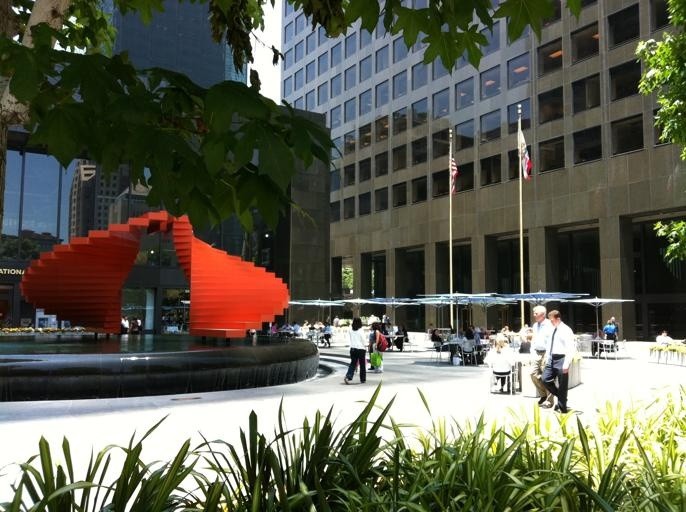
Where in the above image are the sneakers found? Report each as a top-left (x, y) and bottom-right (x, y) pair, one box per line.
(344, 377), (366, 384)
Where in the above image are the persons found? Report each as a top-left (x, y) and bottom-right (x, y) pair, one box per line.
(268, 315), (621, 393)
(655, 330), (675, 350)
(527, 303), (558, 409)
(540, 307), (577, 413)
(119, 306), (190, 335)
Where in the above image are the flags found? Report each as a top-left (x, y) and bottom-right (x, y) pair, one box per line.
(448, 135), (460, 193)
(517, 118), (537, 183)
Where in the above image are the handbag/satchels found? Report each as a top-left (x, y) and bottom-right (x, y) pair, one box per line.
(370, 353), (383, 367)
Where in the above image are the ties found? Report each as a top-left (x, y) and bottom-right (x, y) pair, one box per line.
(550, 327), (558, 356)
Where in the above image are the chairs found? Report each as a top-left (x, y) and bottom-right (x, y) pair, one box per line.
(426, 326), (533, 396)
(363, 326), (419, 353)
(275, 325), (348, 347)
(574, 333), (627, 361)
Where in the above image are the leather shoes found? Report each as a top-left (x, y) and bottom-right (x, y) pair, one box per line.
(538, 396), (568, 413)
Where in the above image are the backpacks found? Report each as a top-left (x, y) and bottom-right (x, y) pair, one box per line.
(378, 333), (387, 352)
